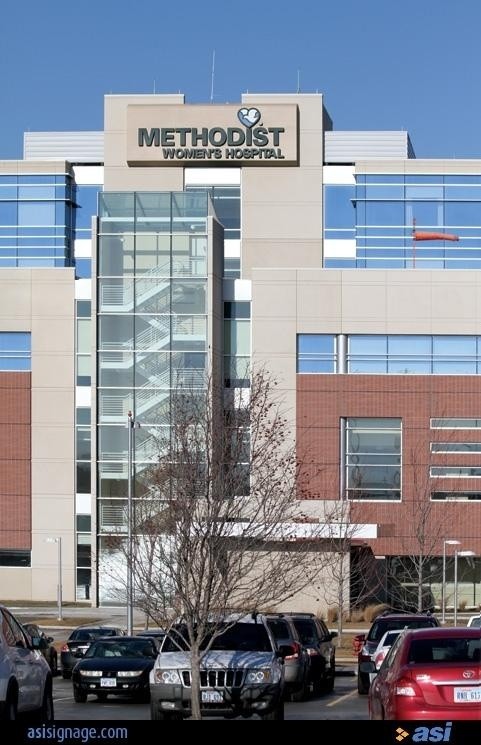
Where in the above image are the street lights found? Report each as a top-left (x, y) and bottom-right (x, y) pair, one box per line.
(44, 537), (63, 622)
(439, 540), (462, 625)
(454, 550), (476, 627)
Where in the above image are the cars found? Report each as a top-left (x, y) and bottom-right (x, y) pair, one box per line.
(359, 615), (481, 720)
(0, 605), (165, 719)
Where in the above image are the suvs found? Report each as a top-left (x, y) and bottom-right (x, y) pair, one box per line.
(353, 610), (441, 695)
(149, 610), (338, 721)
